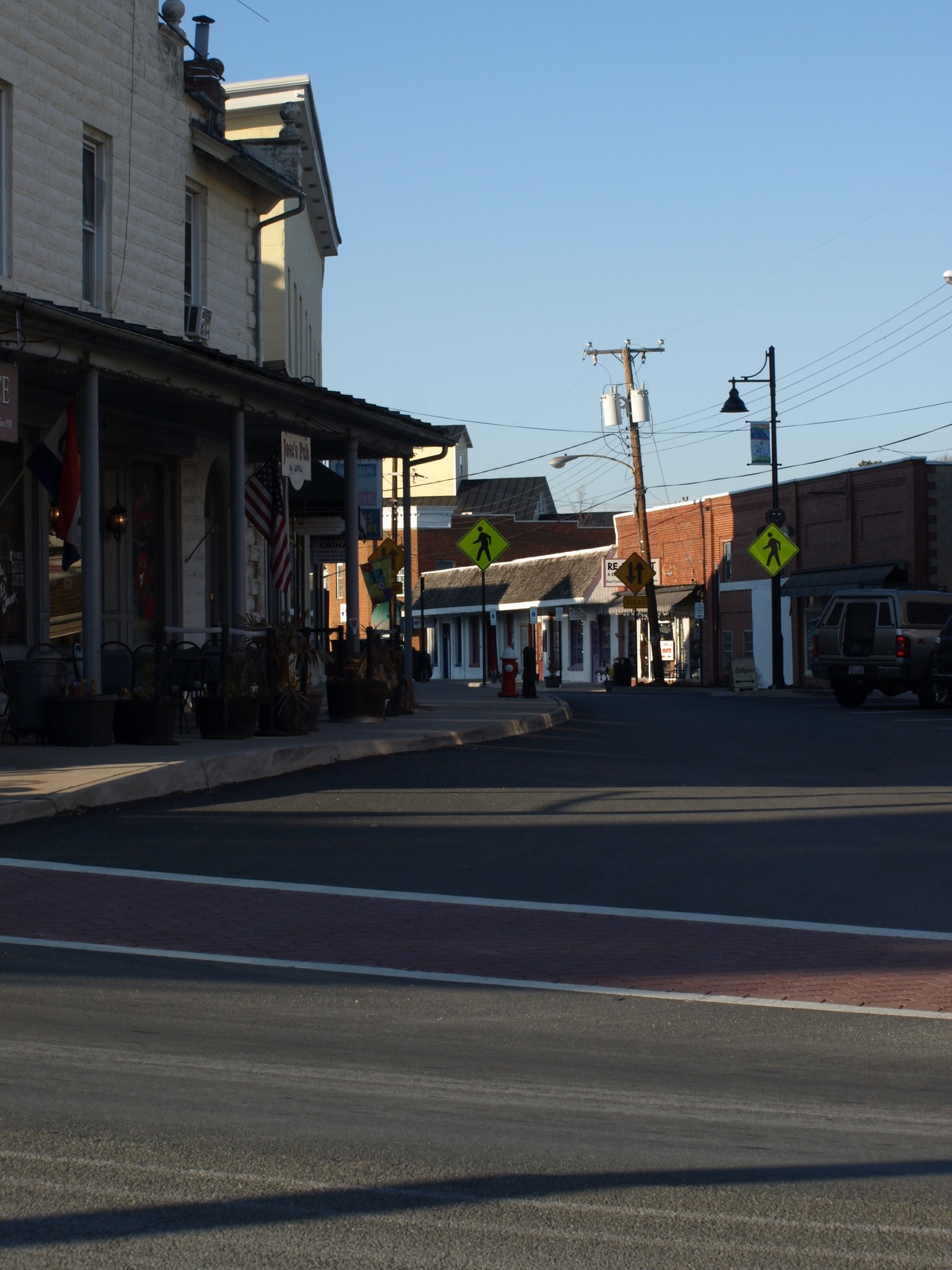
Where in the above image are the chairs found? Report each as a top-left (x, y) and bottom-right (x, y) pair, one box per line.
(668, 661), (687, 685)
(0, 639), (262, 747)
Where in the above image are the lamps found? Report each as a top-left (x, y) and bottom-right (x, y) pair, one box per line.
(105, 496), (130, 545)
(48, 500), (60, 538)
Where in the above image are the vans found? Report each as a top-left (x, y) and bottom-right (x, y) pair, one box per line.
(811, 589), (952, 708)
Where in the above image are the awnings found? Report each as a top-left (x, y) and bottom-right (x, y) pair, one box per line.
(597, 589), (694, 616)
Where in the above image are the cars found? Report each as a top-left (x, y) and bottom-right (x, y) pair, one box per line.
(359, 637), (434, 683)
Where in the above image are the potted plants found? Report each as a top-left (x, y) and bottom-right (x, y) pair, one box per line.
(192, 650), (261, 740)
(326, 624), (403, 718)
(603, 666), (614, 692)
(543, 657), (560, 688)
(42, 677), (118, 751)
(235, 609), (330, 735)
(113, 662), (182, 746)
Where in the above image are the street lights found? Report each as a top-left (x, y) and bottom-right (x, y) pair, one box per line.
(720, 346), (788, 689)
(549, 454), (664, 687)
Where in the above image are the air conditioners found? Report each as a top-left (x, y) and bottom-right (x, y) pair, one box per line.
(184, 303), (213, 341)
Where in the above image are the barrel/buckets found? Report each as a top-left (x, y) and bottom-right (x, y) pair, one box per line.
(5, 659), (66, 734)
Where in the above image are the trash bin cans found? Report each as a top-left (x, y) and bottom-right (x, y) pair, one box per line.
(613, 657), (632, 687)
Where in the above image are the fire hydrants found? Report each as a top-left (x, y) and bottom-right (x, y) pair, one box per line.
(497, 646), (524, 698)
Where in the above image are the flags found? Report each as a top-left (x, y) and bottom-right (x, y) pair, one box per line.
(244, 455), (292, 592)
(27, 399), (81, 571)
